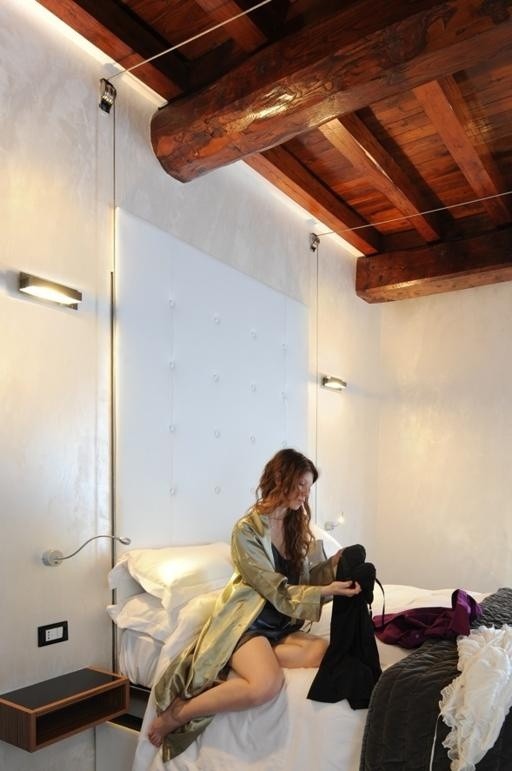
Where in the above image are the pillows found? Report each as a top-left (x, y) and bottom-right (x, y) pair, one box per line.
(106, 593), (169, 643)
(124, 541), (235, 612)
(309, 524), (343, 559)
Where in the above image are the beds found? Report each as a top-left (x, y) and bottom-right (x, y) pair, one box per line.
(106, 544), (512, 771)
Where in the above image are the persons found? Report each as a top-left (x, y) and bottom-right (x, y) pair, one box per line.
(146, 448), (362, 748)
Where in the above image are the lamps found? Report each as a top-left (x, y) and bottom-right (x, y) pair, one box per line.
(42, 534), (131, 567)
(322, 375), (346, 391)
(19, 272), (82, 310)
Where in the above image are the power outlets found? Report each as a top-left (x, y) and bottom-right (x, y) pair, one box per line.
(37, 621), (69, 647)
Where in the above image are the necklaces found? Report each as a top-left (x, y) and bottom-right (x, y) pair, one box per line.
(271, 543), (288, 560)
